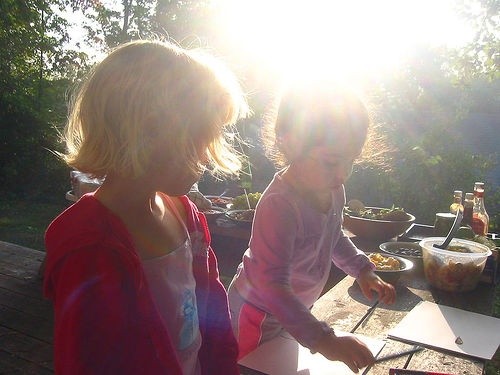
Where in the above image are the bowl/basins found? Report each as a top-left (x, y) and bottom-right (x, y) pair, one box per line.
(192, 195), (258, 226)
(479, 236), (500, 267)
(379, 241), (424, 276)
(420, 236), (492, 293)
(360, 252), (414, 281)
(344, 207), (416, 241)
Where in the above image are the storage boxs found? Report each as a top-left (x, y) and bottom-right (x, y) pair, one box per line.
(69, 171), (106, 197)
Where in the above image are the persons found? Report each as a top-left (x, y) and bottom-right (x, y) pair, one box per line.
(42, 40), (239, 374)
(228, 77), (397, 374)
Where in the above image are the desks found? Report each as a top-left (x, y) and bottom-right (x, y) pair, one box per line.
(209, 224), (378, 261)
(0, 241), (54, 375)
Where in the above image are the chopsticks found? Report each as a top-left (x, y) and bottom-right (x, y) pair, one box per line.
(349, 299), (424, 362)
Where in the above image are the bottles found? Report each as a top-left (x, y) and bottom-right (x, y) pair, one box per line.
(450, 182), (489, 236)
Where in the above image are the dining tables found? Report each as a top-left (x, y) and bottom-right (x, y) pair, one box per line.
(236, 235), (500, 375)
